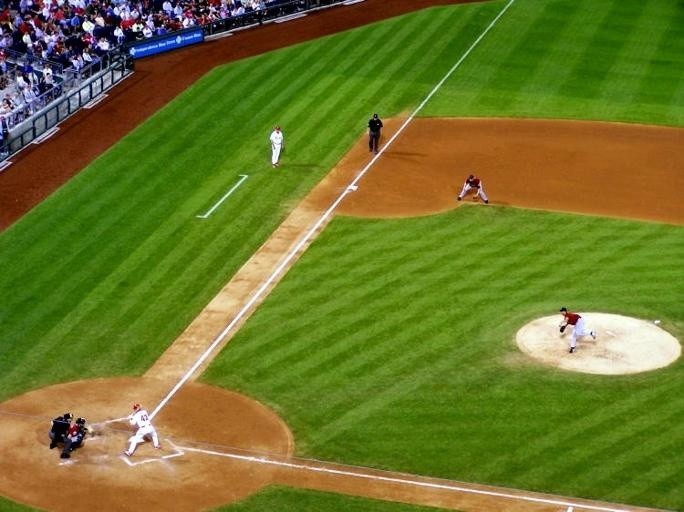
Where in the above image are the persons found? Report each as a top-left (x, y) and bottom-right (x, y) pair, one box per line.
(128, 2), (268, 44)
(269, 126), (283, 168)
(457, 175), (489, 204)
(558, 307), (595, 352)
(0, 1), (129, 133)
(366, 114), (383, 154)
(125, 403), (160, 457)
(48, 413), (88, 458)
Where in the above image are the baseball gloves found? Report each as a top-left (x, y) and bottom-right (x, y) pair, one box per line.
(560, 327), (565, 332)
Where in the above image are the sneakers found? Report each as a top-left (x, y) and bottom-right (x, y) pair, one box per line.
(272, 162), (279, 168)
(369, 148), (377, 154)
(458, 197), (461, 201)
(591, 329), (596, 339)
(50, 443), (57, 449)
(124, 451), (131, 456)
(155, 445), (160, 449)
(569, 347), (575, 353)
(485, 200), (488, 204)
(61, 453), (70, 458)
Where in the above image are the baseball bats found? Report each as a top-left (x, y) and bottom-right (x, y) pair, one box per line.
(105, 418), (129, 423)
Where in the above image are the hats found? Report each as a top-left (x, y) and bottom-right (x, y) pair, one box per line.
(560, 307), (566, 311)
(469, 175), (473, 179)
(374, 114), (377, 118)
(133, 404), (140, 409)
(275, 125), (280, 130)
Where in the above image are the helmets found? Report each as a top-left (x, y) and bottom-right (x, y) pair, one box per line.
(76, 417), (86, 431)
(64, 413), (73, 424)
